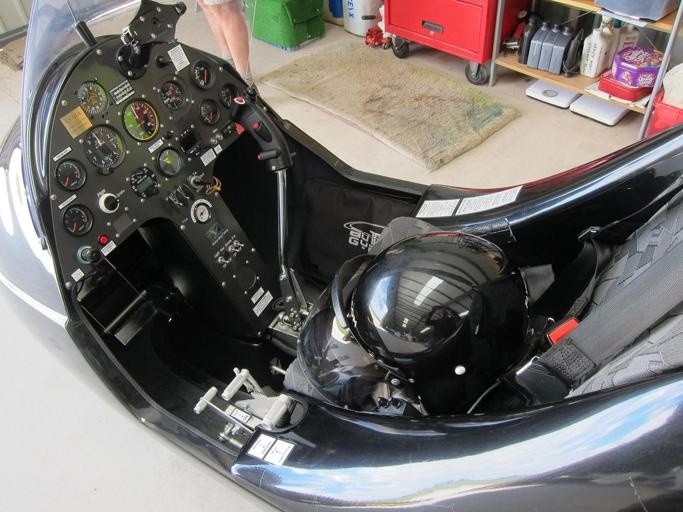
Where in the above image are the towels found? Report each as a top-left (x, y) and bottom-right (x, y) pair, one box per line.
(260, 40), (521, 174)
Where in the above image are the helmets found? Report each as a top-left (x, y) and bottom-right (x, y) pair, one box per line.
(296, 231), (530, 417)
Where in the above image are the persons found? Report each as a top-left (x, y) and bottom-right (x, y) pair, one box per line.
(196, 1), (259, 100)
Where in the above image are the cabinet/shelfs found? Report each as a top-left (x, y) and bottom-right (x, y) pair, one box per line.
(489, 0), (683, 142)
